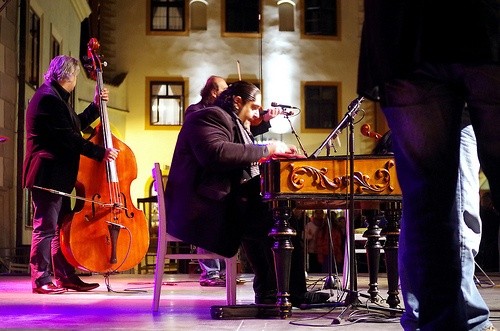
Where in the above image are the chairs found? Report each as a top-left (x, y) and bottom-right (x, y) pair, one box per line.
(150, 163), (237, 311)
(137, 196), (179, 275)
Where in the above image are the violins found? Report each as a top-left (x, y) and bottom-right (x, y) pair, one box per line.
(248, 106), (294, 126)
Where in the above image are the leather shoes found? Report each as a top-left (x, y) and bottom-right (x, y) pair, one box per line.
(57, 276), (100, 291)
(32, 282), (65, 294)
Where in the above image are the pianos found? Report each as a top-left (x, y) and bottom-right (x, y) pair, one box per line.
(260, 152), (405, 320)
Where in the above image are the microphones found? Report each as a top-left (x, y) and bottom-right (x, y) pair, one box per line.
(271, 102), (297, 109)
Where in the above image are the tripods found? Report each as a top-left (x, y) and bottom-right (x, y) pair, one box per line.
(300, 96), (407, 325)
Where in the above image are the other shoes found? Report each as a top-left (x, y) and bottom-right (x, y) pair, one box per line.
(220, 275), (245, 285)
(200, 277), (227, 286)
(292, 291), (330, 306)
(255, 294), (278, 305)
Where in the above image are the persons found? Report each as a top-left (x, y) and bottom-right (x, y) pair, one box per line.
(162, 79), (332, 306)
(183, 76), (284, 287)
(23, 54), (121, 294)
(356, 0), (500, 331)
(288, 208), (346, 272)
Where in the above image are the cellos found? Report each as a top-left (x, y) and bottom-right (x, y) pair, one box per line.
(360, 123), (494, 285)
(59, 37), (150, 294)
